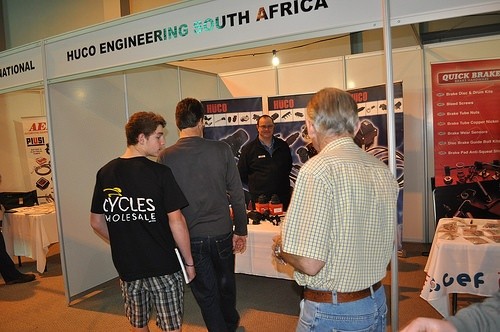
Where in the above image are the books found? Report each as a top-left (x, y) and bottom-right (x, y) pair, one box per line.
(174, 248), (190, 284)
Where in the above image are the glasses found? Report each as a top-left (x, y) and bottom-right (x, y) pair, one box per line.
(259, 125), (274, 129)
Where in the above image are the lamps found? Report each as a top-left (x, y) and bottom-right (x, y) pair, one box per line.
(271, 49), (280, 66)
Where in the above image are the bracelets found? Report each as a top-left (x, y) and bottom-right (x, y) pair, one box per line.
(185, 263), (194, 267)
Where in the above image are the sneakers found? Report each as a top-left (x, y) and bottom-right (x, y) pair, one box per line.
(5, 273), (36, 284)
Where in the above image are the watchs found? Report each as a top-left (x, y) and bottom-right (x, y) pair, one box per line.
(274, 242), (281, 259)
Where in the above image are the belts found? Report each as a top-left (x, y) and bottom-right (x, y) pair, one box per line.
(303, 280), (382, 303)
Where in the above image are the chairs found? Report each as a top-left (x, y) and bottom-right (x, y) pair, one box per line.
(0, 189), (55, 212)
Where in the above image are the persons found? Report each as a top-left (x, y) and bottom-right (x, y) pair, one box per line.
(237, 114), (292, 212)
(0, 203), (35, 284)
(401, 287), (500, 332)
(272, 88), (399, 332)
(156, 98), (249, 332)
(90, 111), (196, 332)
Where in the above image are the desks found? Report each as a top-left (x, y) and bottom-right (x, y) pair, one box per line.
(3, 203), (61, 274)
(417, 216), (500, 319)
(231, 211), (294, 280)
